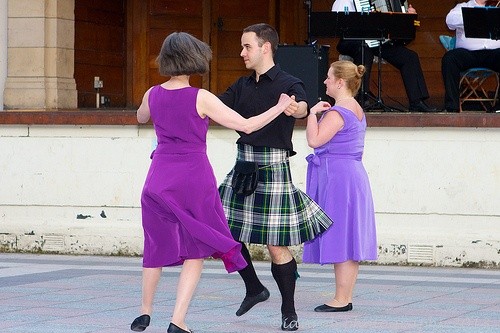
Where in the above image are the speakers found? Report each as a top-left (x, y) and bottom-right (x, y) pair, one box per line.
(274, 44), (330, 110)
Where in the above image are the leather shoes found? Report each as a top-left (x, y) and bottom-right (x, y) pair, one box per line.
(409, 100), (437, 113)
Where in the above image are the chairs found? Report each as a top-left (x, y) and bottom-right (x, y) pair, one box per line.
(441, 34), (499, 111)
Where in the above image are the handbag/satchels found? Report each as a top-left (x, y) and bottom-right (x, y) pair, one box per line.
(232, 160), (258, 197)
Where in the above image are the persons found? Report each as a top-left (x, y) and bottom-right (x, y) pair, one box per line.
(131, 31), (295, 333)
(442, 0), (500, 113)
(331, 0), (446, 112)
(217, 23), (334, 331)
(302, 60), (378, 313)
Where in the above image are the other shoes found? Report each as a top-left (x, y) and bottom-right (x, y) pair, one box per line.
(281, 313), (298, 331)
(131, 314), (150, 331)
(167, 323), (194, 333)
(314, 303), (353, 312)
(236, 288), (270, 316)
(442, 108), (460, 113)
